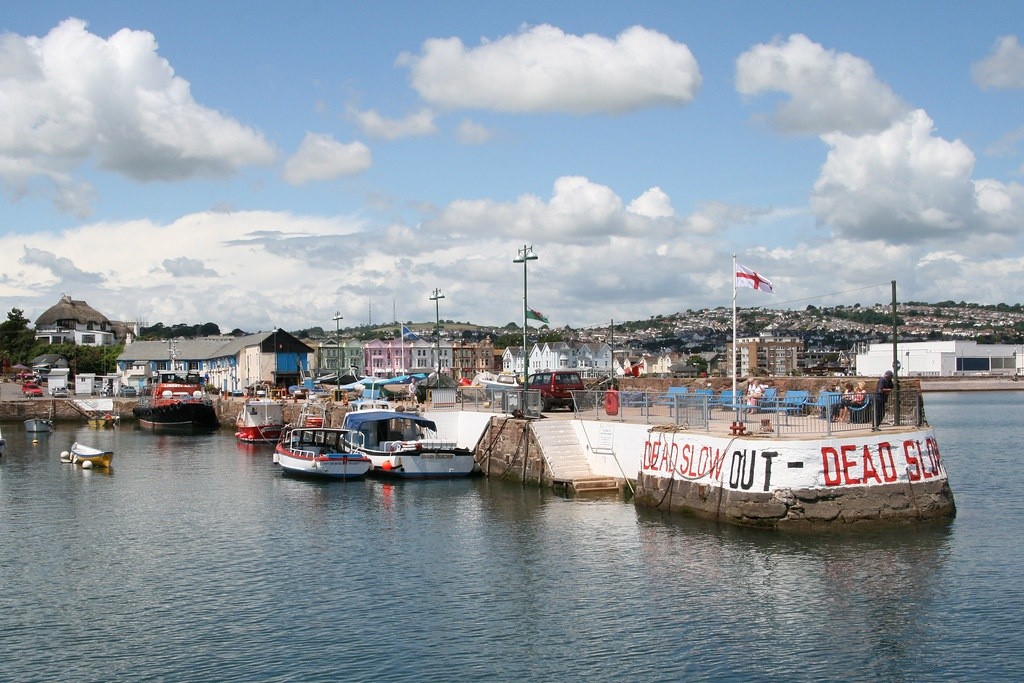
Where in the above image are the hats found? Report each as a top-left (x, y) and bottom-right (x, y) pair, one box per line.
(884, 370), (893, 376)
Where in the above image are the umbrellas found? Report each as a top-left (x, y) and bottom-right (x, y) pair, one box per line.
(11, 364), (29, 369)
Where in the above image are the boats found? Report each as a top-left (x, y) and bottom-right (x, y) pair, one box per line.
(63, 395), (120, 427)
(273, 402), (373, 479)
(0, 429), (7, 458)
(233, 388), (286, 442)
(132, 397), (221, 434)
(24, 418), (52, 432)
(337, 408), (474, 479)
(70, 441), (114, 467)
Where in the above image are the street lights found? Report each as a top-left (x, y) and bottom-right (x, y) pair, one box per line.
(332, 310), (344, 402)
(512, 243), (539, 419)
(429, 288), (445, 389)
(271, 325), (278, 388)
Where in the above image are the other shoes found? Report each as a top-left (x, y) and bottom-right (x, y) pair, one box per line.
(872, 427), (881, 431)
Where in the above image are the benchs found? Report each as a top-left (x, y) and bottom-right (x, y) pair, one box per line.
(658, 387), (688, 408)
(814, 392), (843, 421)
(780, 390), (811, 416)
(691, 389), (715, 407)
(749, 388), (778, 412)
(832, 393), (870, 422)
(720, 389), (744, 412)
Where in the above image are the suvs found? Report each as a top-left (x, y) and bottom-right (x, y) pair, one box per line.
(119, 386), (136, 397)
(21, 383), (42, 397)
(513, 370), (586, 413)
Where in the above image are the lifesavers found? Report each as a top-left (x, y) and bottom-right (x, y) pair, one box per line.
(169, 403), (175, 411)
(390, 440), (403, 451)
(305, 418), (323, 428)
(105, 414), (112, 419)
(401, 441), (422, 451)
(158, 404), (167, 413)
(134, 409), (152, 418)
(176, 401), (183, 410)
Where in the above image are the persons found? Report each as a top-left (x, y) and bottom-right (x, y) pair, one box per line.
(289, 430), (299, 441)
(409, 378), (418, 407)
(833, 381), (867, 422)
(20, 371), (26, 385)
(744, 378), (765, 414)
(872, 370), (893, 431)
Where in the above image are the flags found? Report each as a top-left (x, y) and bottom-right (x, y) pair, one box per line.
(526, 302), (550, 324)
(403, 325), (419, 340)
(735, 263), (776, 294)
(616, 362), (644, 378)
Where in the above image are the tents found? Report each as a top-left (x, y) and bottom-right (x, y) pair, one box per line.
(317, 372), (472, 399)
